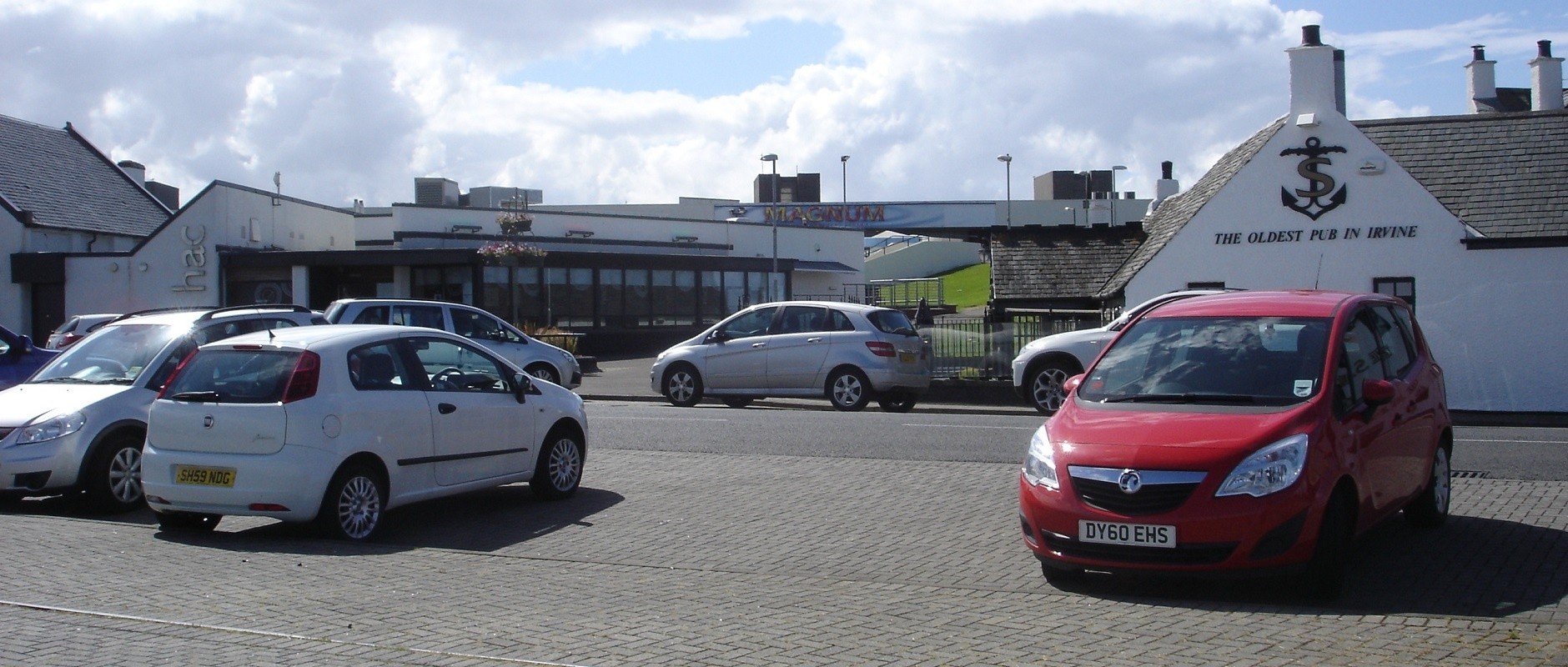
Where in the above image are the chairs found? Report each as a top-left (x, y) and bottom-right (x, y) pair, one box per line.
(1177, 323), (1225, 394)
(359, 353), (395, 388)
(782, 314), (802, 332)
(1287, 324), (1330, 396)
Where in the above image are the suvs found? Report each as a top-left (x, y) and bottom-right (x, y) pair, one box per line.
(323, 297), (583, 392)
(0, 296), (333, 514)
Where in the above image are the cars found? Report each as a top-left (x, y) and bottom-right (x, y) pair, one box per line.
(141, 323), (588, 546)
(1008, 285), (1449, 579)
(650, 299), (932, 412)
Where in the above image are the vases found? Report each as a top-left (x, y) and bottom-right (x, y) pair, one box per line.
(499, 223), (517, 235)
(514, 220), (532, 231)
(521, 254), (545, 268)
(482, 255), (499, 267)
(499, 254), (521, 267)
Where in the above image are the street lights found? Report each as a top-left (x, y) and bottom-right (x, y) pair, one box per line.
(761, 154), (778, 302)
(997, 154), (1012, 229)
(1112, 165), (1128, 227)
(1065, 207), (1076, 226)
(841, 155), (850, 203)
(1079, 171), (1089, 226)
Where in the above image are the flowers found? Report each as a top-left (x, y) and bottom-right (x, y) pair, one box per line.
(476, 211), (549, 258)
